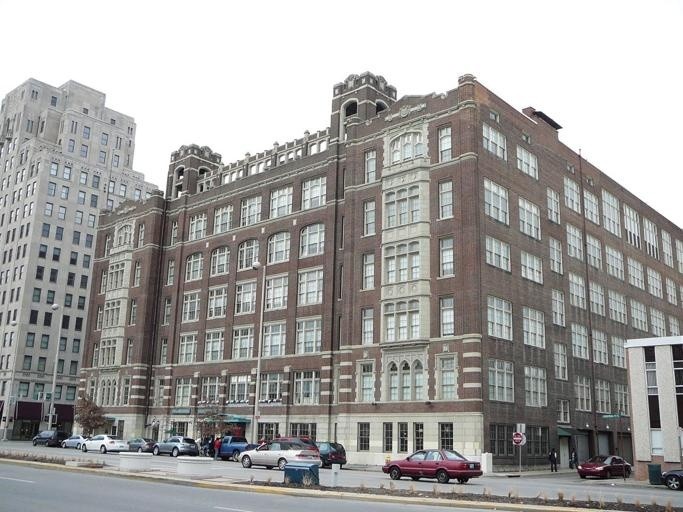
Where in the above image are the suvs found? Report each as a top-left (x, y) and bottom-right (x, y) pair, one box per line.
(153, 436), (199, 457)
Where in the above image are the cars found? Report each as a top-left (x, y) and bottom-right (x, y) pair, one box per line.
(80, 435), (129, 453)
(381, 448), (482, 484)
(61, 435), (91, 450)
(577, 454), (632, 479)
(661, 470), (683, 490)
(128, 438), (156, 454)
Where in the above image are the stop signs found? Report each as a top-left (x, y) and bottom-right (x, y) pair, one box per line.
(513, 433), (523, 445)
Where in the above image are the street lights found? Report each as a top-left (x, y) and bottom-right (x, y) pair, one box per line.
(48, 304), (64, 430)
(1, 321), (17, 440)
(251, 261), (266, 445)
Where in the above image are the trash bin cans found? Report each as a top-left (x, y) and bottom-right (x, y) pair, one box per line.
(284, 462), (319, 485)
(647, 463), (662, 485)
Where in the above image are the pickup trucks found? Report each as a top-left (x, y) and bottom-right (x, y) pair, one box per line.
(215, 435), (259, 462)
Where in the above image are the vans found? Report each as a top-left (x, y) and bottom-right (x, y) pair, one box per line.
(238, 437), (346, 471)
(33, 430), (67, 448)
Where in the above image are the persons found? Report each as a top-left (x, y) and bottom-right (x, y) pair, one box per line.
(548, 448), (559, 472)
(202, 434), (222, 460)
(569, 448), (578, 468)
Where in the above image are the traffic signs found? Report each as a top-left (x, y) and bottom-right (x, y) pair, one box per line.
(602, 413), (620, 418)
